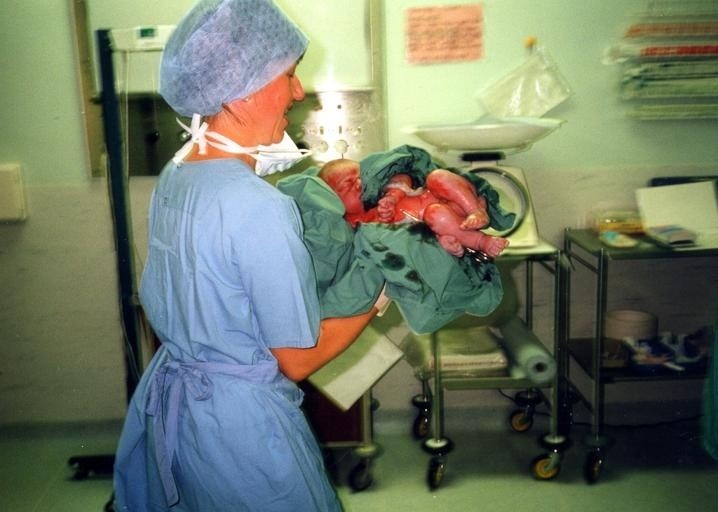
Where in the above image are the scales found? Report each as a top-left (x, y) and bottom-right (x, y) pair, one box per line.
(408, 121), (553, 249)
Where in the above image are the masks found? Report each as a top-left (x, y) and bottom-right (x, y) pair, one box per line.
(255, 132), (316, 178)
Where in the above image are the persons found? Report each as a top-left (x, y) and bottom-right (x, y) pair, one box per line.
(113, 1), (394, 512)
(317, 158), (510, 258)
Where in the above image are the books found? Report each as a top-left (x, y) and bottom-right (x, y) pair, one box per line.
(649, 225), (699, 248)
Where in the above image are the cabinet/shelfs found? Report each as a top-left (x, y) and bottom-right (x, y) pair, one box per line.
(412, 225), (717, 488)
